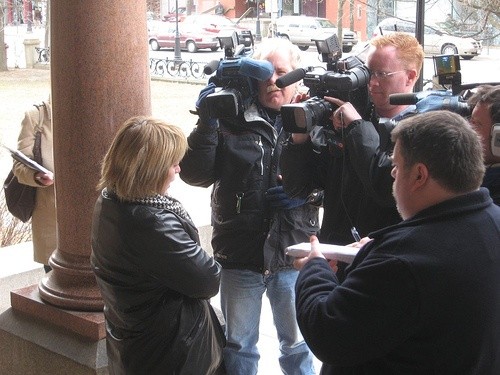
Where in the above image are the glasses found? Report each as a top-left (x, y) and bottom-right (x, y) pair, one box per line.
(370, 70), (407, 79)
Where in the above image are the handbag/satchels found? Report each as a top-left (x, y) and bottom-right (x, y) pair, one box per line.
(4, 103), (45, 222)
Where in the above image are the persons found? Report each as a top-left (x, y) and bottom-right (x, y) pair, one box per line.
(280, 33), (424, 375)
(179, 36), (325, 375)
(90, 115), (227, 375)
(466, 84), (500, 208)
(34, 6), (43, 28)
(3, 94), (58, 273)
(294, 110), (500, 375)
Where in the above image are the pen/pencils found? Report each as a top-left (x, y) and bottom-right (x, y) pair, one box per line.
(352, 227), (360, 242)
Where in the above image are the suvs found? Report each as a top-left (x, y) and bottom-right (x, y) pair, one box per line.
(274, 16), (358, 53)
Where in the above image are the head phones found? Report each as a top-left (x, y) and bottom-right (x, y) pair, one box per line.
(490, 123), (500, 157)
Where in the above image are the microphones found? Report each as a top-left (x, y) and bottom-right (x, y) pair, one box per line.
(389, 94), (418, 105)
(203, 60), (220, 75)
(275, 68), (306, 88)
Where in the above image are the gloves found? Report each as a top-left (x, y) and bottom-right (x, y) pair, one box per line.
(195, 82), (218, 128)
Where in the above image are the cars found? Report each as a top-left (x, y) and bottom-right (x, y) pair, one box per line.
(165, 6), (185, 23)
(371, 17), (483, 60)
(184, 13), (257, 49)
(147, 23), (220, 53)
